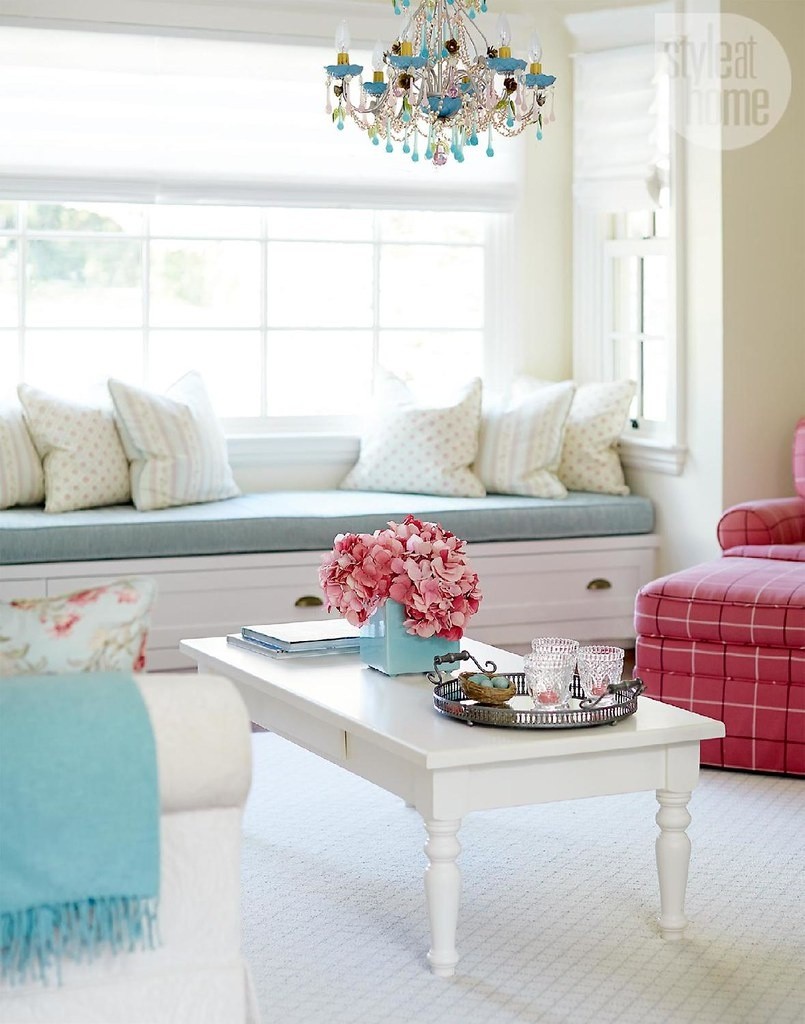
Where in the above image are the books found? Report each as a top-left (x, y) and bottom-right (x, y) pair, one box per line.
(227, 618), (360, 660)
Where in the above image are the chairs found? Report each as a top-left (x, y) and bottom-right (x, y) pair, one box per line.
(716, 415), (805, 563)
(0, 674), (251, 1024)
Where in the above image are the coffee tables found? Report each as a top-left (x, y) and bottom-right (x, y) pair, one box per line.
(178, 633), (726, 978)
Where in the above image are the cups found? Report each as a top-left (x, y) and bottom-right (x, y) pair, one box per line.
(524, 652), (577, 709)
(577, 646), (625, 707)
(531, 637), (581, 674)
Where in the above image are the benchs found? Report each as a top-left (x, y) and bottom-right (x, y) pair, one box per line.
(0, 417), (659, 670)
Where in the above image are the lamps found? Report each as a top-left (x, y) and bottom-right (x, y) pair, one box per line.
(321, 1), (557, 167)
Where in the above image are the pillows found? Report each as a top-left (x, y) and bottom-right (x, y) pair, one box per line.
(0, 577), (158, 674)
(554, 379), (636, 496)
(339, 377), (486, 497)
(470, 379), (576, 498)
(106, 370), (241, 511)
(16, 383), (129, 514)
(0, 411), (45, 509)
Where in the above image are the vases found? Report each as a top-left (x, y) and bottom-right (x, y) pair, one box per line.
(359, 598), (460, 678)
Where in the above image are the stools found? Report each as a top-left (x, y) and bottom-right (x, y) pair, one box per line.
(632, 556), (804, 779)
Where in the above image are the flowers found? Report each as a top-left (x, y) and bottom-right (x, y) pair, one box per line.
(316, 512), (483, 641)
